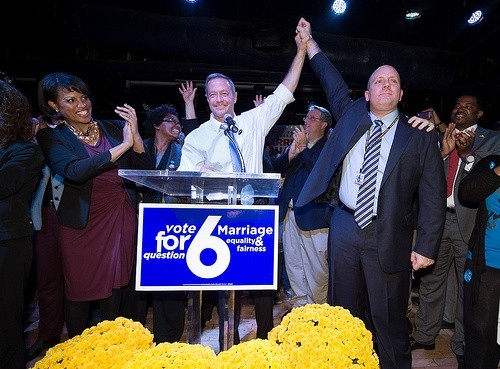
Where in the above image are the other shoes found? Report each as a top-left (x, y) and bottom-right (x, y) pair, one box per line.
(26, 335), (61, 362)
(440, 320), (455, 333)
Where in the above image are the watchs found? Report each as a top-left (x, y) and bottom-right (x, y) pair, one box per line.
(461, 150), (476, 164)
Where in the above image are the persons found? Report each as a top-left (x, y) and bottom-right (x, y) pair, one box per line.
(176, 31), (312, 351)
(37, 73), (148, 338)
(294, 17), (448, 369)
(457, 154), (500, 369)
(0, 80), (500, 369)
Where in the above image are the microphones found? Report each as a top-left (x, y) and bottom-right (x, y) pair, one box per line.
(223, 113), (238, 133)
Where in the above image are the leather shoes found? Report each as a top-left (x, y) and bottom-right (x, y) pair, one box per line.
(456, 355), (466, 369)
(409, 336), (436, 350)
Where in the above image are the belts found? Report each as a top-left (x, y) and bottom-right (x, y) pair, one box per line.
(446, 207), (456, 214)
(288, 207), (294, 211)
(337, 198), (378, 220)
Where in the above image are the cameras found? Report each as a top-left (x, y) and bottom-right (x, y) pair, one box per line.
(417, 111), (432, 120)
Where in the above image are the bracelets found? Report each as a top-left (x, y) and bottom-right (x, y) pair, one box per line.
(435, 121), (444, 129)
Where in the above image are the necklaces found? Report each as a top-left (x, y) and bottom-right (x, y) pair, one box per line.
(64, 120), (99, 143)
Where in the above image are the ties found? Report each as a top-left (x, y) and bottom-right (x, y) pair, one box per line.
(220, 124), (245, 172)
(353, 119), (384, 230)
(446, 147), (460, 198)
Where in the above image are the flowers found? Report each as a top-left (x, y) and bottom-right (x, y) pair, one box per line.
(28, 302), (380, 369)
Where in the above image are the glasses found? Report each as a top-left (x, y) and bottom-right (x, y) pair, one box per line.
(162, 117), (184, 128)
(302, 116), (321, 121)
